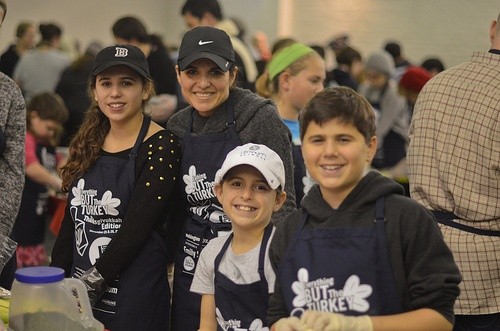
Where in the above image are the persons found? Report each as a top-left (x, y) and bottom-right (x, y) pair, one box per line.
(188, 141), (291, 331)
(164, 23), (304, 331)
(267, 87), (464, 331)
(0, 0), (500, 331)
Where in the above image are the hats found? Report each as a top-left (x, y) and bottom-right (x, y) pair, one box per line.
(88, 44), (152, 83)
(219, 143), (285, 192)
(400, 66), (433, 93)
(177, 26), (234, 72)
(366, 50), (397, 77)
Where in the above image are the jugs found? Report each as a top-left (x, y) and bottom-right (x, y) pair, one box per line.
(9, 266), (93, 331)
